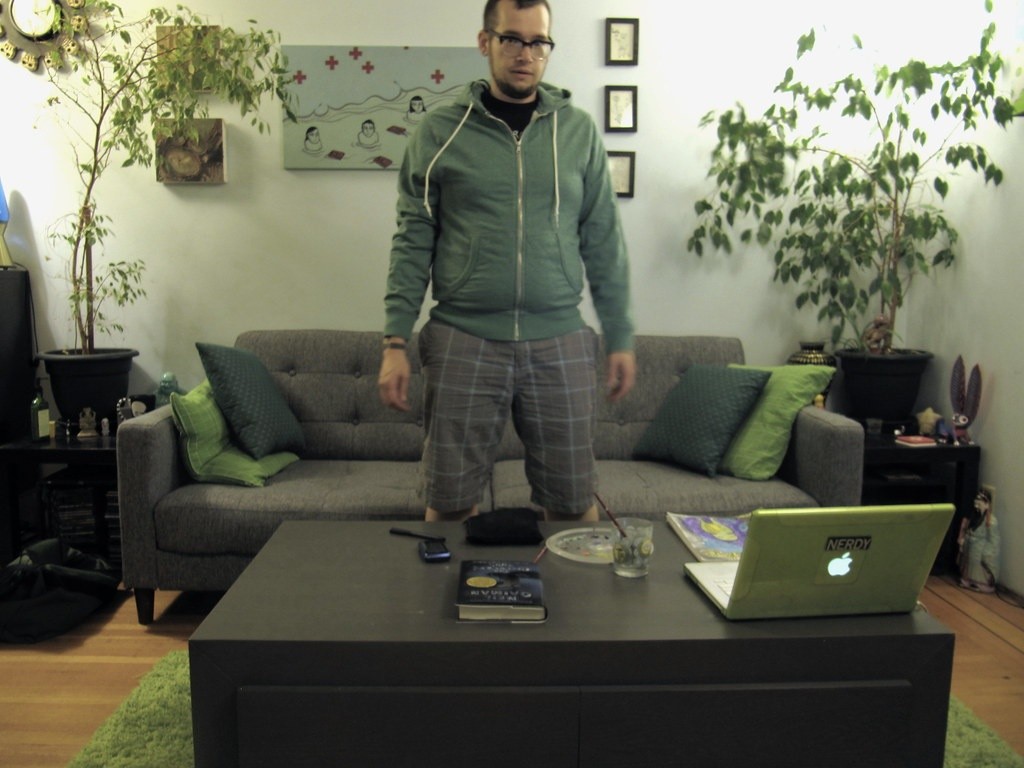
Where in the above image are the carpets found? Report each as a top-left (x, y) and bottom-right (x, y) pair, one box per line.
(70, 622), (1024, 767)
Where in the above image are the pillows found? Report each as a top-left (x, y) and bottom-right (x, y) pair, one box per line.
(169, 376), (297, 488)
(196, 341), (309, 457)
(633, 363), (771, 476)
(716, 364), (837, 479)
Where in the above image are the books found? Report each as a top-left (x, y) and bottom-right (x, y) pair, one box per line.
(104, 490), (122, 565)
(665, 507), (769, 561)
(54, 503), (96, 546)
(455, 560), (549, 624)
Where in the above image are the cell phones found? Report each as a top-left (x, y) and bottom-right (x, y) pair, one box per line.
(420, 540), (452, 562)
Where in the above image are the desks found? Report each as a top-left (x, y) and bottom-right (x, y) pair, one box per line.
(186, 518), (953, 766)
(0, 439), (121, 567)
(866, 432), (981, 574)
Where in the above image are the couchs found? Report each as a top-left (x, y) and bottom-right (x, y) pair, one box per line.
(119, 329), (865, 625)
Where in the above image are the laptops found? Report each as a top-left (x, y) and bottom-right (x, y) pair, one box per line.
(684, 503), (955, 619)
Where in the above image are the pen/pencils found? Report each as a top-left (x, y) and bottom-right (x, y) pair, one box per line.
(389, 527), (446, 542)
(532, 546), (549, 562)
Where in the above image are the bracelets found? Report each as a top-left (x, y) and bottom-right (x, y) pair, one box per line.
(382, 343), (406, 351)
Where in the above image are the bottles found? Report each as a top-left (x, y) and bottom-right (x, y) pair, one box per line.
(31, 386), (51, 443)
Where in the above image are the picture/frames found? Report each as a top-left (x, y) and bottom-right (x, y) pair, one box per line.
(607, 151), (635, 197)
(605, 18), (639, 65)
(604, 86), (638, 131)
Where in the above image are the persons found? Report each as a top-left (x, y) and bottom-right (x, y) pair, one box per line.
(958, 489), (1000, 593)
(153, 372), (186, 408)
(377, 0), (635, 522)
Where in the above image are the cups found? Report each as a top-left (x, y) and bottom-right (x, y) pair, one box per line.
(612, 517), (654, 578)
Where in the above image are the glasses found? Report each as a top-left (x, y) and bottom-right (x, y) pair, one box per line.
(487, 29), (553, 60)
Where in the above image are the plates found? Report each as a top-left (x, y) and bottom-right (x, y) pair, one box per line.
(546, 527), (614, 565)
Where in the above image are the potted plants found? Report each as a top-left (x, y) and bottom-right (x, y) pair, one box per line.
(29, 1), (301, 422)
(687, 1), (1024, 434)
